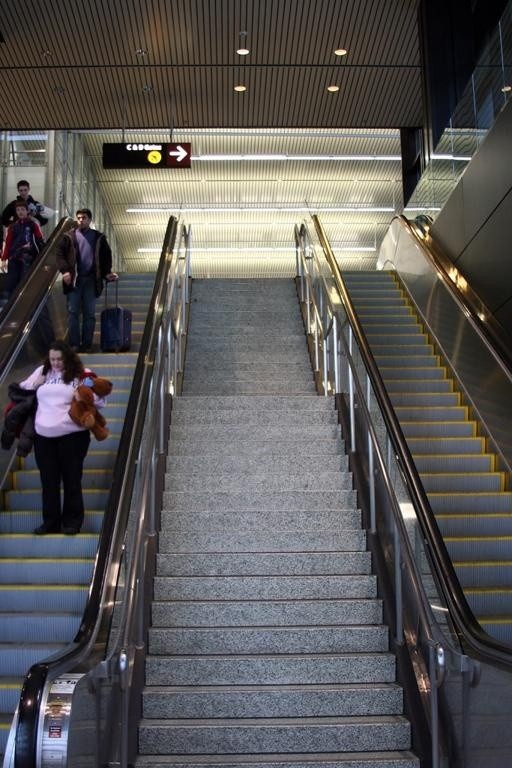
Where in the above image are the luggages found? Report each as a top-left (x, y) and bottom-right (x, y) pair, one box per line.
(101, 278), (131, 353)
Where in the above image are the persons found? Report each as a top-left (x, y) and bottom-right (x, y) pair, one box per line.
(55, 208), (118, 353)
(1, 180), (48, 227)
(21, 341), (106, 534)
(1, 201), (43, 296)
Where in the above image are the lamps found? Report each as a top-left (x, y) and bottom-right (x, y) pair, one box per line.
(236, 25), (249, 56)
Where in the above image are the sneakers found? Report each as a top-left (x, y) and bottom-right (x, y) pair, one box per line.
(61, 526), (79, 534)
(70, 346), (93, 353)
(34, 524), (61, 535)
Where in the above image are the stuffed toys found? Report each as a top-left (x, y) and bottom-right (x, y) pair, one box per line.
(69, 371), (113, 441)
(0, 381), (40, 459)
